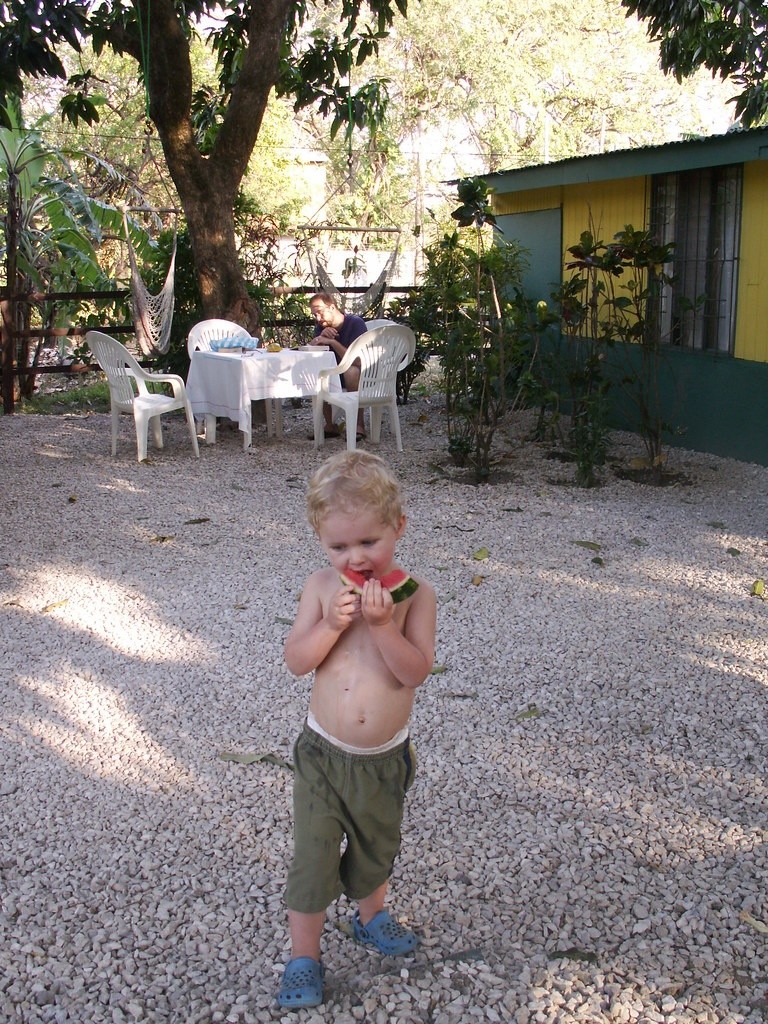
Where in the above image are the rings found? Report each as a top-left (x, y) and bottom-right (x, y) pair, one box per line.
(316, 339), (319, 342)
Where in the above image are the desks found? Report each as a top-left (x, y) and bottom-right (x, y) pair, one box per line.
(186, 348), (343, 449)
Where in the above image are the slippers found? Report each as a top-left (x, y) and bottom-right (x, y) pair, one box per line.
(306, 429), (340, 439)
(345, 432), (367, 443)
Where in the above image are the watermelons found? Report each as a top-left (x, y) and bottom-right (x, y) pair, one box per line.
(339, 568), (418, 604)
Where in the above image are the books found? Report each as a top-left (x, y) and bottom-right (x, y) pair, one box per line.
(290, 343), (329, 351)
(217, 347), (242, 353)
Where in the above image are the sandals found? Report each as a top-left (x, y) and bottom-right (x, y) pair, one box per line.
(278, 956), (325, 1009)
(352, 908), (417, 955)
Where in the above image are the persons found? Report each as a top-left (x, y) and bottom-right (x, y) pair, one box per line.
(281, 448), (437, 1007)
(309, 294), (370, 439)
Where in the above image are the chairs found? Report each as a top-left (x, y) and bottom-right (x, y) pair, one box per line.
(85, 331), (200, 462)
(187, 319), (273, 444)
(364, 318), (416, 371)
(313, 324), (416, 453)
